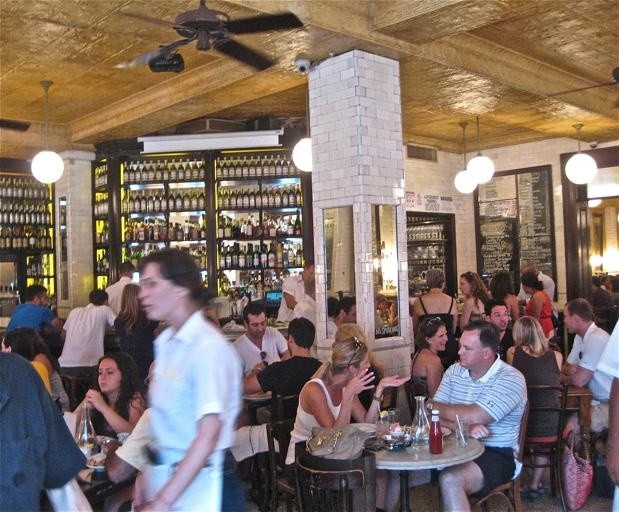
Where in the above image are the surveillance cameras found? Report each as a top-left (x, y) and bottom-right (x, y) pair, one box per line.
(295, 60), (311, 75)
(589, 141), (600, 149)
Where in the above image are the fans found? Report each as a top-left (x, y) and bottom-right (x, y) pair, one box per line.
(111, 0), (304, 74)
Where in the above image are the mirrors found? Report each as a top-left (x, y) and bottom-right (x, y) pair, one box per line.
(321, 205), (358, 340)
(370, 202), (402, 340)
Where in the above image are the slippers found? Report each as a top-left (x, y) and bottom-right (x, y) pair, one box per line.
(538, 483), (545, 496)
(520, 486), (538, 498)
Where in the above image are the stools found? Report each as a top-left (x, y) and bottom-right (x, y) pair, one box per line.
(59, 372), (96, 410)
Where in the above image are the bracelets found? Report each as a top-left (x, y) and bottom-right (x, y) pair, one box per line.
(371, 395), (382, 402)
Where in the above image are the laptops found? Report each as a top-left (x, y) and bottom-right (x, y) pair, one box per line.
(265, 292), (282, 309)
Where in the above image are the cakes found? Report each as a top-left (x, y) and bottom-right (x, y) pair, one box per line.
(390, 423), (402, 433)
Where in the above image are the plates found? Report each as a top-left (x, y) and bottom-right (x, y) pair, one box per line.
(86, 453), (107, 473)
(349, 422), (377, 436)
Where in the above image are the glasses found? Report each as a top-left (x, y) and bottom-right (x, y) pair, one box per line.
(578, 351), (582, 359)
(261, 352), (268, 367)
(425, 316), (441, 329)
(347, 336), (363, 369)
(137, 277), (172, 287)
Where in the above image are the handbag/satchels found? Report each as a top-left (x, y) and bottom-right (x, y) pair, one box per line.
(560, 431), (594, 511)
(306, 426), (366, 460)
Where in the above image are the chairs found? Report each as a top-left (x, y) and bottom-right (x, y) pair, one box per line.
(266, 420), (300, 511)
(525, 383), (571, 512)
(404, 377), (422, 422)
(294, 440), (377, 512)
(276, 393), (303, 420)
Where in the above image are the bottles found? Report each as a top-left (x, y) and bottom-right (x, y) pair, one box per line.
(94, 163), (108, 188)
(228, 270), (263, 316)
(219, 240), (302, 270)
(141, 159), (149, 184)
(121, 191), (205, 213)
(410, 395), (431, 452)
(129, 160), (135, 185)
(429, 409), (443, 454)
(218, 184), (301, 210)
(96, 248), (110, 275)
(125, 213), (206, 242)
(75, 403), (98, 460)
(95, 219), (109, 244)
(155, 159), (162, 183)
(216, 152), (301, 181)
(191, 156), (199, 182)
(199, 156), (206, 182)
(0, 225), (51, 251)
(122, 245), (208, 271)
(407, 215), (444, 239)
(170, 158), (177, 183)
(404, 427), (413, 447)
(0, 176), (50, 200)
(264, 270), (304, 291)
(203, 275), (208, 287)
(147, 159), (155, 184)
(102, 277), (107, 293)
(184, 157), (192, 182)
(94, 192), (109, 217)
(217, 209), (302, 240)
(1, 198), (52, 225)
(134, 160), (141, 184)
(162, 158), (169, 182)
(178, 157), (184, 183)
(123, 160), (129, 185)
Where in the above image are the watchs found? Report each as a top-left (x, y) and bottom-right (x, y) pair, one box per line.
(424, 398), (434, 412)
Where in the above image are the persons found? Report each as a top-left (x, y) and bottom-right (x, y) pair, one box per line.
(131, 248), (243, 511)
(593, 318), (619, 512)
(462, 272), (492, 329)
(507, 315), (563, 499)
(101, 360), (157, 511)
(518, 258), (555, 328)
(114, 283), (163, 379)
(290, 276), (316, 319)
(409, 315), (450, 414)
(243, 318), (324, 436)
(486, 300), (515, 363)
(285, 335), (411, 512)
(7, 284), (62, 337)
(59, 287), (118, 411)
(232, 301), (292, 426)
(72, 352), (146, 436)
(274, 257), (314, 320)
(105, 261), (136, 316)
(375, 320), (526, 511)
(590, 276), (615, 319)
(0, 332), (52, 404)
(1, 353), (92, 512)
(490, 273), (519, 322)
(13, 327), (70, 413)
(522, 270), (555, 339)
(414, 269), (460, 366)
(325, 296), (342, 320)
(331, 324), (382, 412)
(562, 298), (614, 451)
(327, 296), (356, 347)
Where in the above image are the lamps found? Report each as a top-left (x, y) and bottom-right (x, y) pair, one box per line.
(31, 81), (65, 185)
(566, 122), (599, 186)
(466, 115), (495, 184)
(290, 80), (312, 173)
(454, 121), (479, 196)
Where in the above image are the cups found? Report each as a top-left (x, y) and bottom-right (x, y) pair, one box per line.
(455, 420), (469, 448)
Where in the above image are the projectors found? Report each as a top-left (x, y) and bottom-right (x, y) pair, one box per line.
(149, 52), (185, 72)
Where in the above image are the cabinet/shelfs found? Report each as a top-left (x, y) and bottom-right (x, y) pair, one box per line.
(0, 156), (54, 251)
(92, 140), (113, 304)
(112, 135), (214, 329)
(213, 147), (314, 328)
